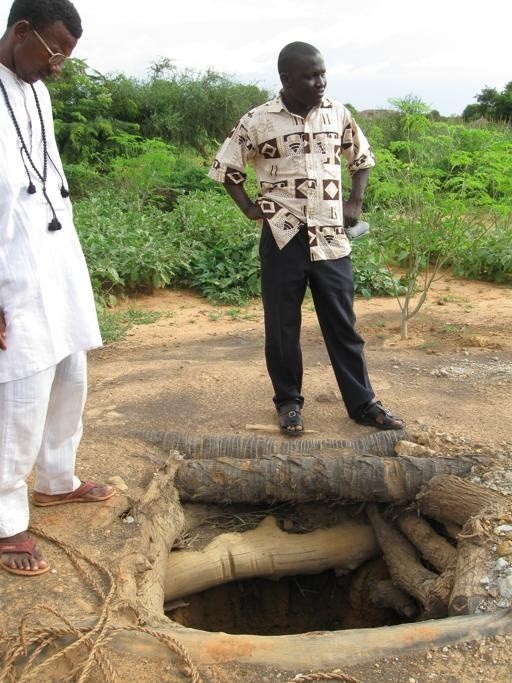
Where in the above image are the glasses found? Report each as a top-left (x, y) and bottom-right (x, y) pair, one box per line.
(33, 30), (69, 64)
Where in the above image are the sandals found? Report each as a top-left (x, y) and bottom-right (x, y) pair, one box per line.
(279, 404), (304, 435)
(356, 399), (405, 429)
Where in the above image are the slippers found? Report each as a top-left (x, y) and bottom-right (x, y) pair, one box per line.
(32, 480), (116, 506)
(0, 535), (50, 575)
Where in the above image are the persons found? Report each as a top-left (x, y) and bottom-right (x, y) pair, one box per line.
(1, 2), (118, 578)
(205, 41), (406, 434)
